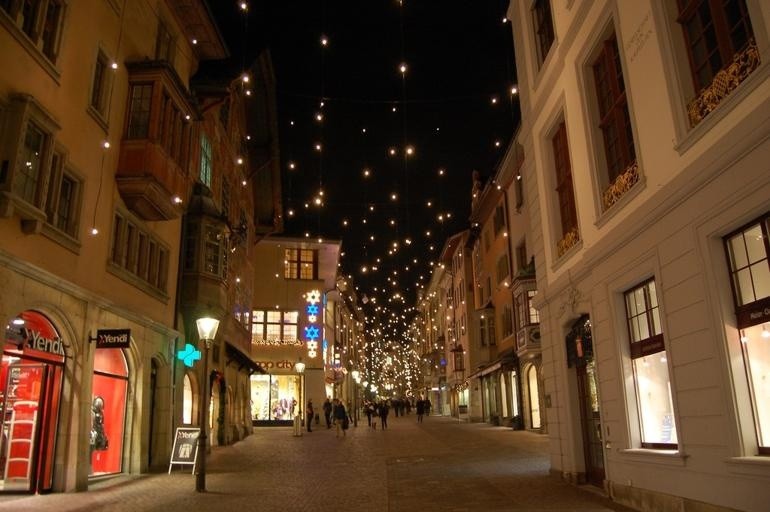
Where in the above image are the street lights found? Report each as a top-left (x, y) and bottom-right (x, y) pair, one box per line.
(351, 370), (361, 427)
(194, 313), (221, 493)
(295, 362), (306, 415)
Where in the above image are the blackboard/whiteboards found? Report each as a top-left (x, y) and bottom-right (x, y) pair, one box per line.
(170, 428), (201, 464)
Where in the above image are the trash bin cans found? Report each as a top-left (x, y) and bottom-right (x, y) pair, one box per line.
(293, 416), (302, 437)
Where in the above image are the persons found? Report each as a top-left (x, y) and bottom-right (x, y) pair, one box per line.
(305, 393), (433, 439)
(89, 394), (109, 477)
